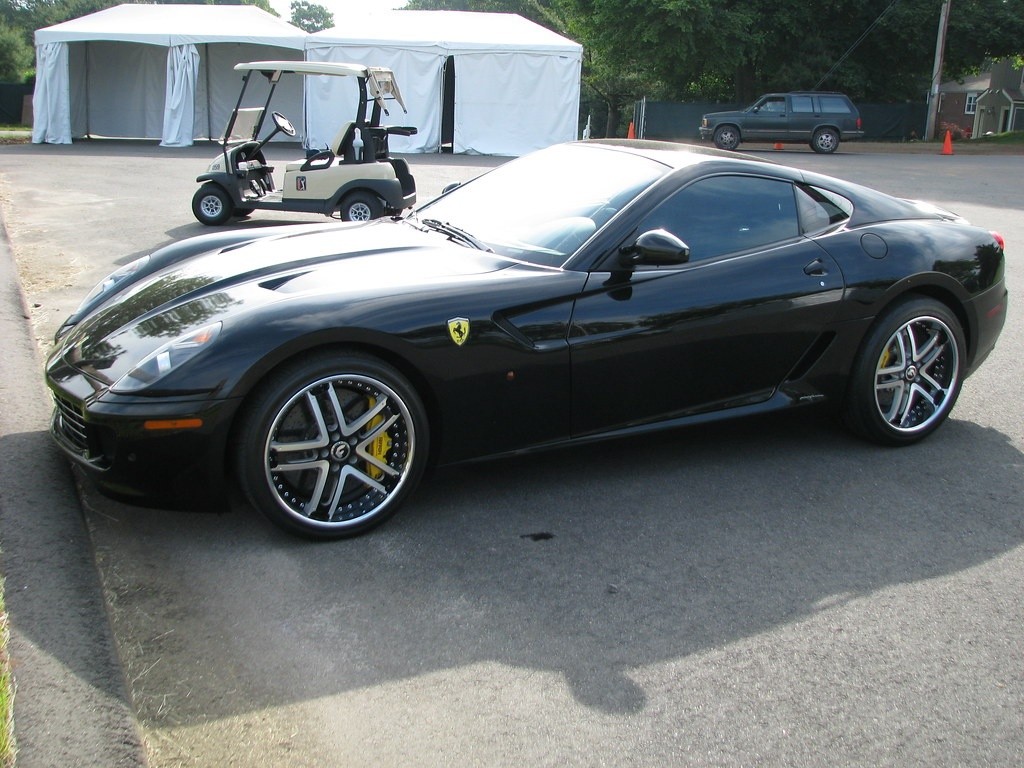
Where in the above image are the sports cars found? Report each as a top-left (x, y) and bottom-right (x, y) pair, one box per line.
(41, 132), (1005, 542)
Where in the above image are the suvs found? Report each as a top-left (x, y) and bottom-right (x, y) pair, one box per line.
(696, 93), (862, 152)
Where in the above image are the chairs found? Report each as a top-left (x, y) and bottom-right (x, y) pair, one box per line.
(286, 119), (359, 172)
